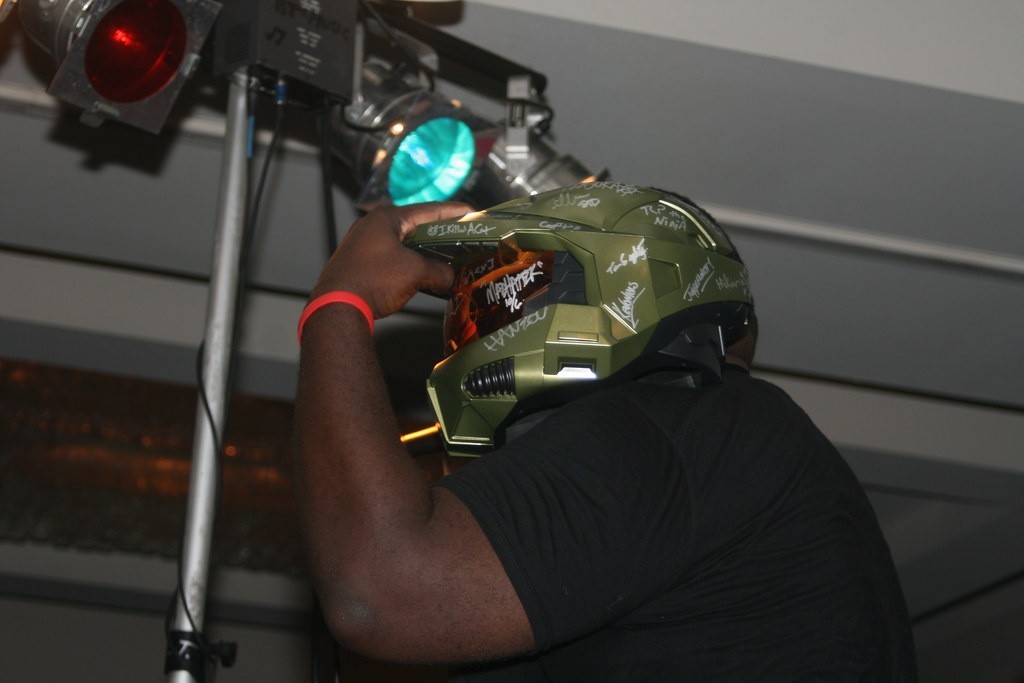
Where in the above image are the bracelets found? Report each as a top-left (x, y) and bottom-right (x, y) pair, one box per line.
(297, 291), (375, 346)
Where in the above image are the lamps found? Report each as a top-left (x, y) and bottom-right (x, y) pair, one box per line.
(16, 0), (224, 135)
(480, 122), (610, 205)
(313, 65), (479, 207)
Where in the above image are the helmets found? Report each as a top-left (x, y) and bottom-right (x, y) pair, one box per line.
(400, 180), (759, 459)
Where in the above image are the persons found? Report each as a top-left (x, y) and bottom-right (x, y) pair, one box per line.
(294, 182), (918, 683)
